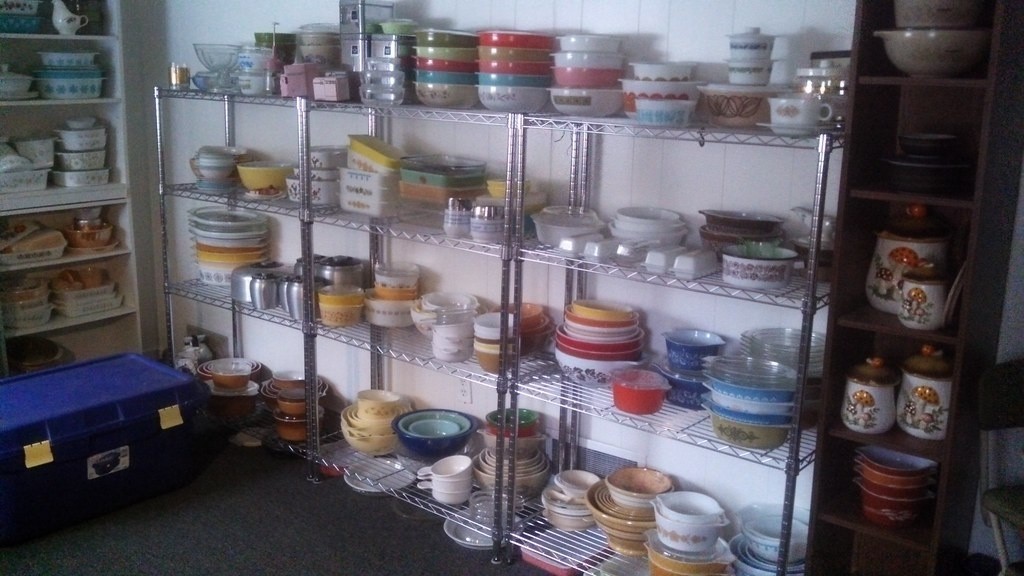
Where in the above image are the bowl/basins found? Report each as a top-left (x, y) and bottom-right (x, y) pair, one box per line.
(0, 278), (56, 329)
(50, 116), (111, 187)
(873, 28), (991, 79)
(0, 169), (52, 195)
(852, 445), (940, 530)
(31, 50), (108, 100)
(10, 137), (58, 170)
(188, 11), (849, 575)
(878, 133), (974, 195)
(63, 208), (113, 248)
(0, 0), (46, 33)
(0, 64), (32, 94)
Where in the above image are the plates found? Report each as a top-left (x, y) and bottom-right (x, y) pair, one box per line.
(0, 91), (40, 100)
(443, 509), (522, 550)
(66, 238), (120, 254)
(941, 255), (966, 332)
(344, 459), (418, 497)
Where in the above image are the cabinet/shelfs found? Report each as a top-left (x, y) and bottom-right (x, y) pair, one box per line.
(0, 0), (144, 381)
(153, 0), (844, 576)
(803, 0), (1024, 576)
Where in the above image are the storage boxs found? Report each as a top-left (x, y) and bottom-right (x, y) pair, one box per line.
(0, 353), (210, 548)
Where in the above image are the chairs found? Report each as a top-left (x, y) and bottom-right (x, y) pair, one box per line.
(968, 359), (1024, 576)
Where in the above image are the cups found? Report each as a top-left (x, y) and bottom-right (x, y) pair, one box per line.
(167, 62), (191, 90)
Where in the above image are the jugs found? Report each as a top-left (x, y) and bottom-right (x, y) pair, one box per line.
(840, 204), (954, 442)
(51, 0), (89, 36)
